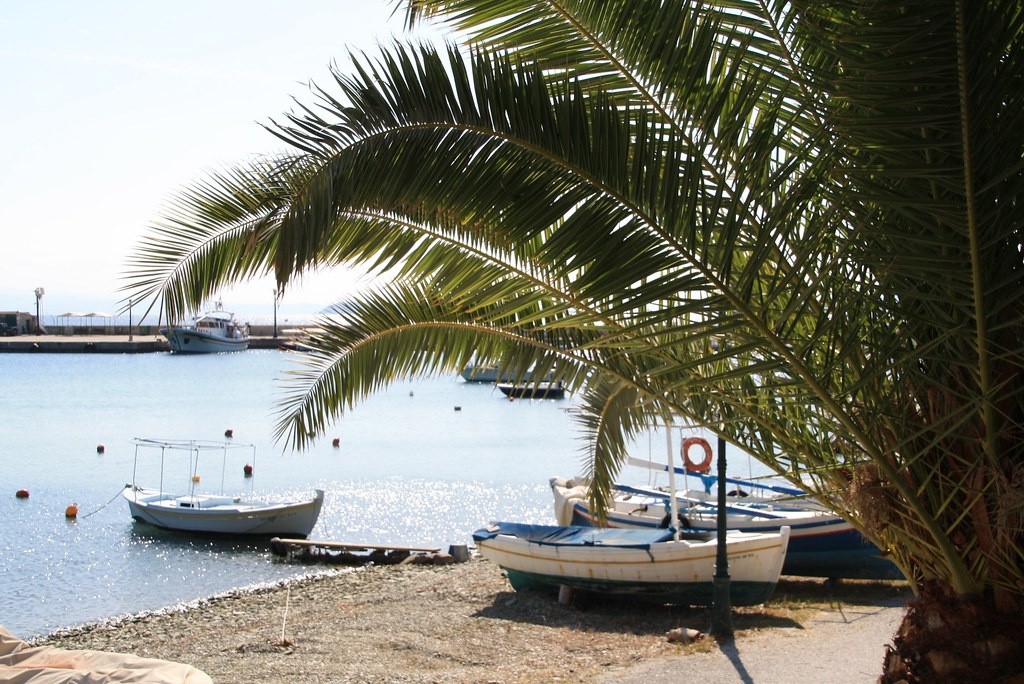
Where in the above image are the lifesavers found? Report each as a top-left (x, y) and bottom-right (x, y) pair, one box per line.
(679, 435), (714, 473)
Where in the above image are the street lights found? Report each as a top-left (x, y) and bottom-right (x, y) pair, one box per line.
(128, 299), (138, 341)
(34, 288), (45, 335)
(273, 288), (283, 338)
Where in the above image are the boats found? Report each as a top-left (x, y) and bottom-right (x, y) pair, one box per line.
(548, 476), (908, 581)
(156, 299), (251, 355)
(496, 380), (565, 398)
(472, 525), (795, 608)
(122, 436), (326, 540)
(455, 360), (570, 385)
